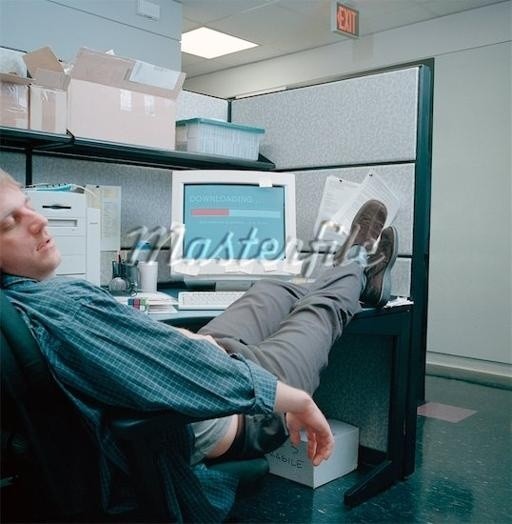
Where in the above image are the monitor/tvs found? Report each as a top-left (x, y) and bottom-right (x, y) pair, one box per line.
(171, 171), (297, 289)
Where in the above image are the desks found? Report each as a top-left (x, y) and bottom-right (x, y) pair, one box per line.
(107, 282), (415, 510)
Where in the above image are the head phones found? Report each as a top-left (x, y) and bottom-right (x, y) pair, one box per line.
(109, 275), (137, 296)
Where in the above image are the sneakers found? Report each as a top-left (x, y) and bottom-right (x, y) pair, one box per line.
(333, 199), (399, 308)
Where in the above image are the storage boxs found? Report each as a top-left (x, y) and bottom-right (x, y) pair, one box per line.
(269, 419), (360, 492)
(0, 43), (266, 163)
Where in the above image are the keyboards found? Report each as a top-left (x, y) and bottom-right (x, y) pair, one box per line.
(177, 290), (247, 311)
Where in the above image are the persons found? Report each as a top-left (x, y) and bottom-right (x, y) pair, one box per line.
(0, 161), (399, 464)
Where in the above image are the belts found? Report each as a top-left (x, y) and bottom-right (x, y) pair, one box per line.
(204, 412), (246, 462)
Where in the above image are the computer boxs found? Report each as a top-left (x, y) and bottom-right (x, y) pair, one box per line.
(27, 188), (87, 276)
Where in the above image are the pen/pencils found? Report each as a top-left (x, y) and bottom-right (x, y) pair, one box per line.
(118, 255), (139, 276)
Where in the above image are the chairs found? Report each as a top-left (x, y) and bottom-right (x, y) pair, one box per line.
(0, 294), (269, 524)
